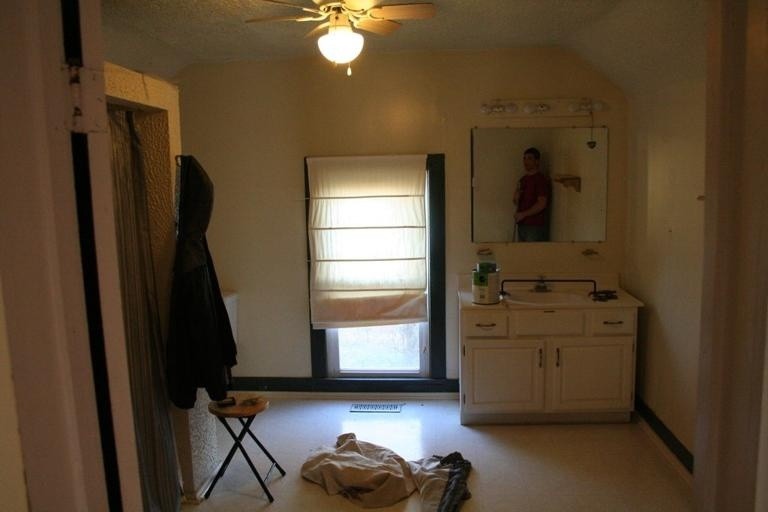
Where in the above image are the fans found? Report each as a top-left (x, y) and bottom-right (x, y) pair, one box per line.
(245, 1), (435, 37)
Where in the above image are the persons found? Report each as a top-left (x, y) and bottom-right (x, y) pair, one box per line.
(511, 145), (553, 241)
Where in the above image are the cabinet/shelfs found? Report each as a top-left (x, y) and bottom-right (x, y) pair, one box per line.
(459, 309), (645, 424)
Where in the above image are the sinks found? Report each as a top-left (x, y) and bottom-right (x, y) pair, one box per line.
(503, 290), (586, 307)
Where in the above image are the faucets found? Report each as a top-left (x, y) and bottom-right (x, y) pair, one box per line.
(534, 275), (546, 292)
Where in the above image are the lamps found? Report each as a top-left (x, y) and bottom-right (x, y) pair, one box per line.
(308, 1), (369, 73)
(480, 97), (604, 119)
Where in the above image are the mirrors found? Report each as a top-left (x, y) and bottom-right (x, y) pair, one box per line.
(470, 127), (609, 246)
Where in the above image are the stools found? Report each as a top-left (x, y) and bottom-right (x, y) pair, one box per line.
(199, 396), (288, 501)
